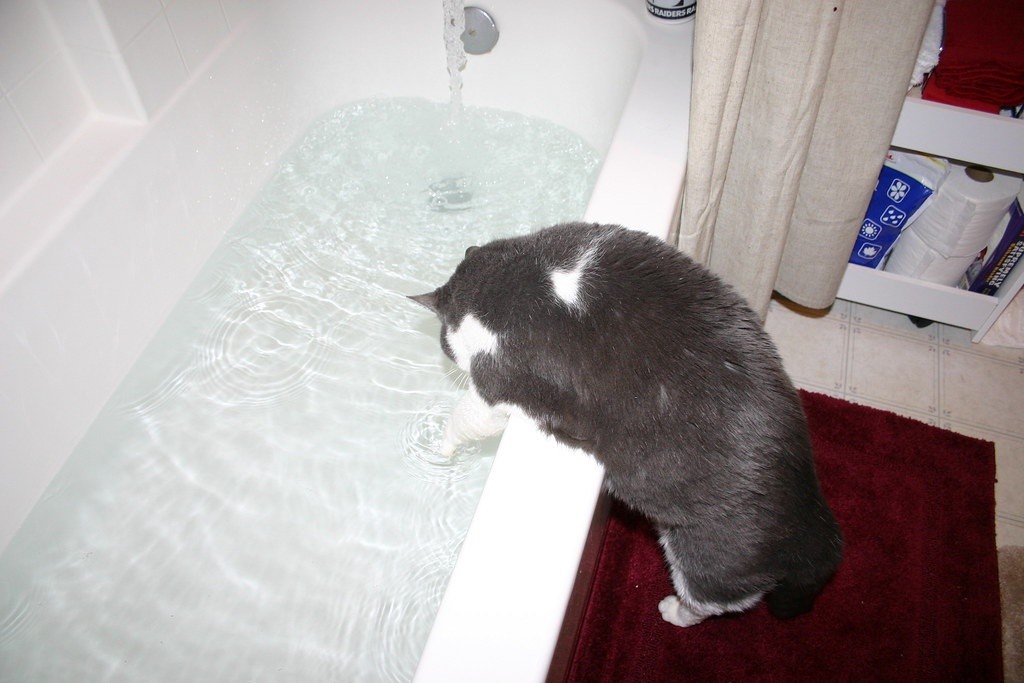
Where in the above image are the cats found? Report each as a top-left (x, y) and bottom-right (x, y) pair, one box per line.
(406, 222), (841, 627)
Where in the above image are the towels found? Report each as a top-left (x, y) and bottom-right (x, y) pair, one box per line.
(908, 0), (1024, 119)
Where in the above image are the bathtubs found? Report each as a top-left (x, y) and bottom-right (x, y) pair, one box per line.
(1, 0), (693, 683)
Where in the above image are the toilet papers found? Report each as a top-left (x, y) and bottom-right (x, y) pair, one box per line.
(882, 162), (1023, 287)
